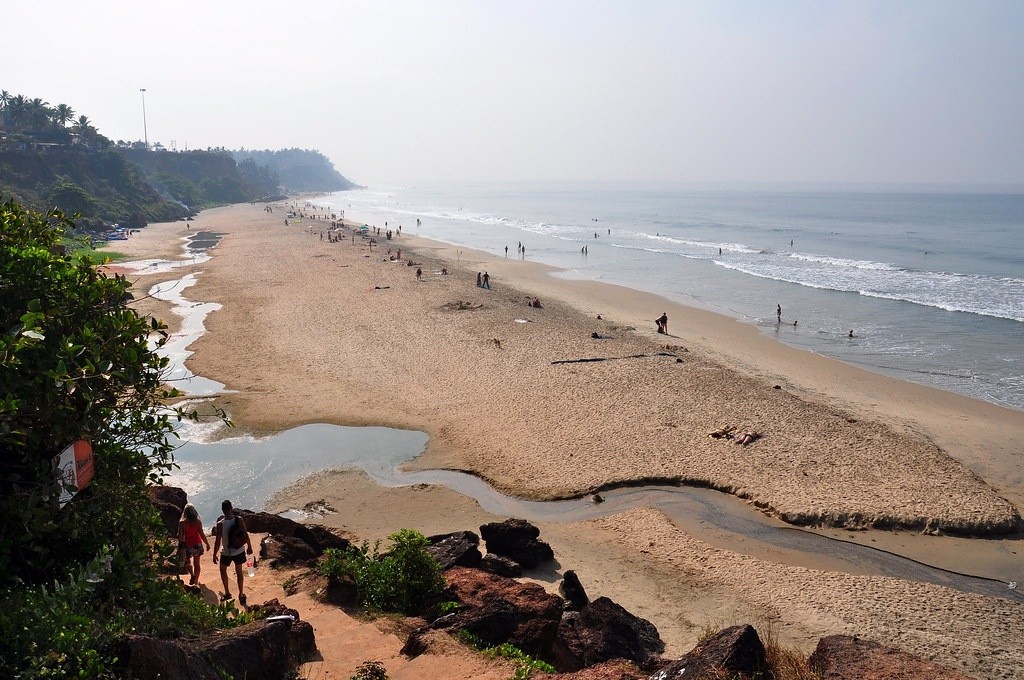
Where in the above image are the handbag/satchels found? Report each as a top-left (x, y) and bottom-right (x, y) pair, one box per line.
(226, 515), (248, 549)
(176, 521), (187, 561)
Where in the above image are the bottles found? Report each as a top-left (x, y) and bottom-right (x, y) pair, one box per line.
(246, 553), (255, 578)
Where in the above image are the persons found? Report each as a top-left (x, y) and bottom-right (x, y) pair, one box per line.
(707, 425), (737, 438)
(794, 321), (797, 326)
(477, 272), (481, 287)
(735, 431), (763, 445)
(213, 500), (253, 603)
(482, 272), (490, 288)
(178, 503), (210, 585)
(416, 267), (422, 281)
(777, 305), (781, 324)
(130, 231), (133, 238)
(661, 313), (668, 335)
(849, 330), (854, 337)
(261, 191), (612, 266)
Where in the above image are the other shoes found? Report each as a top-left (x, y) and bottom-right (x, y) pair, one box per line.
(220, 594), (232, 601)
(188, 576), (196, 585)
(238, 593), (247, 606)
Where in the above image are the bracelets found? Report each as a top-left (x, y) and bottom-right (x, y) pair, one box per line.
(206, 545), (210, 548)
